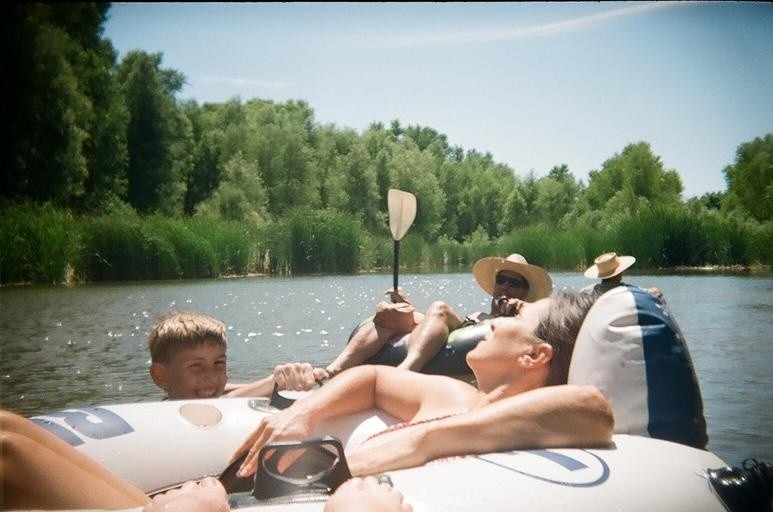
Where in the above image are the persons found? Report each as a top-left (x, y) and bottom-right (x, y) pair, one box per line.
(0, 288), (616, 512)
(147, 316), (325, 399)
(580, 252), (663, 300)
(142, 475), (413, 512)
(325, 253), (552, 372)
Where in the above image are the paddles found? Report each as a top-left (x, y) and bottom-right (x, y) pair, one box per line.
(388, 188), (418, 292)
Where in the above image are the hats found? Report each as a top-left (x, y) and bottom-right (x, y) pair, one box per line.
(584, 252), (636, 279)
(473, 253), (553, 302)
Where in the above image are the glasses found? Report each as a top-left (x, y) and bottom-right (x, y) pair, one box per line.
(495, 275), (528, 289)
